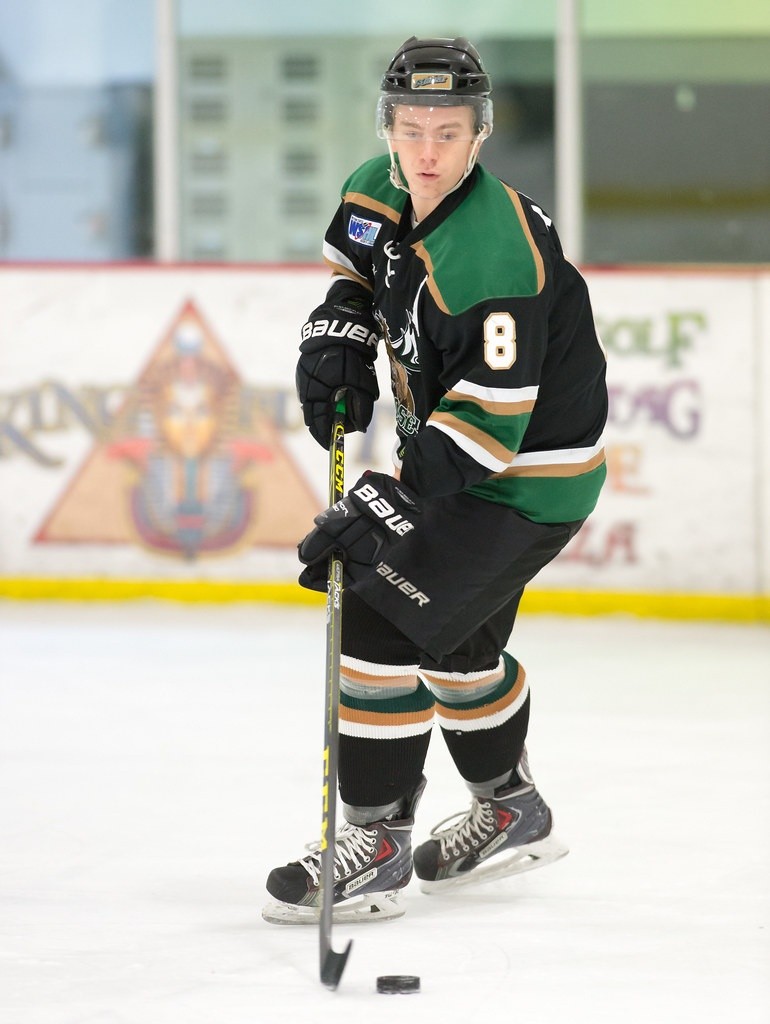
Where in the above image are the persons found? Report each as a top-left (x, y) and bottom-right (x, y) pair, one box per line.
(260, 36), (608, 926)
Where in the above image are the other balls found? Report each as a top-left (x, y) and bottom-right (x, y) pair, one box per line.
(376, 975), (421, 994)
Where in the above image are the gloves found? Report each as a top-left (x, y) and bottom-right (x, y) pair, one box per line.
(295, 316), (380, 452)
(297, 470), (426, 594)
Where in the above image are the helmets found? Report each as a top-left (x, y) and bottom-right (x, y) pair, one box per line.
(380, 35), (494, 134)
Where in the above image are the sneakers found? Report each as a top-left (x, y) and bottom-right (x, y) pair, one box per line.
(261, 773), (428, 923)
(413, 744), (569, 896)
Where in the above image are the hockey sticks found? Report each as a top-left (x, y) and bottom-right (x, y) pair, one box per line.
(311, 397), (352, 992)
